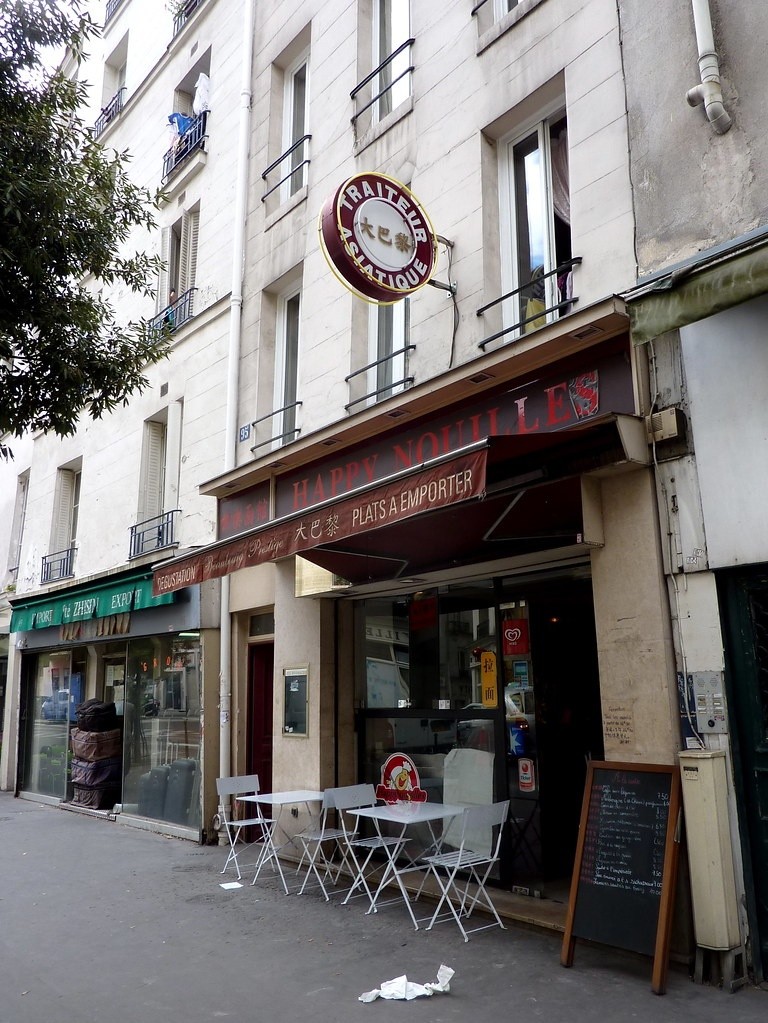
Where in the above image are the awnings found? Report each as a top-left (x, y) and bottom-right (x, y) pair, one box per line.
(151, 429), (592, 599)
(9, 571), (179, 634)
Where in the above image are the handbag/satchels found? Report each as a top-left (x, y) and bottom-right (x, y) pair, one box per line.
(71, 784), (121, 810)
(75, 698), (117, 732)
(71, 758), (122, 787)
(71, 727), (121, 762)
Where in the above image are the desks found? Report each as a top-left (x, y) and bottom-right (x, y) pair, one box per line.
(345, 801), (468, 931)
(235, 790), (325, 896)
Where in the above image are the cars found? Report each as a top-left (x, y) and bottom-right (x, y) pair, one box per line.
(39, 688), (70, 721)
(456, 684), (536, 748)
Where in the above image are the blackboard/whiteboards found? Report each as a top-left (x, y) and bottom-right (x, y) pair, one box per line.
(568, 760), (682, 959)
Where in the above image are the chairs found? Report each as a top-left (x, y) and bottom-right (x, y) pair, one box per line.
(334, 784), (414, 912)
(293, 782), (363, 902)
(420, 801), (510, 942)
(215, 775), (277, 881)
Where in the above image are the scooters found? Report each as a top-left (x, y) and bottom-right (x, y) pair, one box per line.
(141, 692), (162, 717)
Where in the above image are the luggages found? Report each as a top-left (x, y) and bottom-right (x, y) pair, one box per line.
(138, 757), (200, 827)
